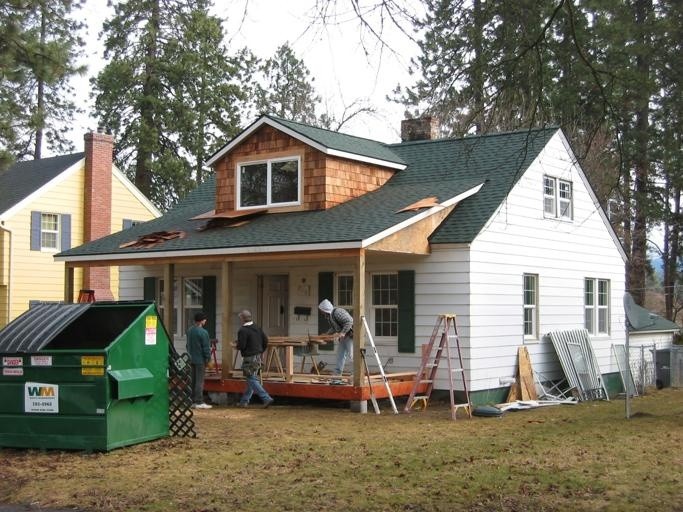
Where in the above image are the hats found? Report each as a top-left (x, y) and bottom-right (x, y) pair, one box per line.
(195, 312), (208, 321)
(239, 310), (252, 320)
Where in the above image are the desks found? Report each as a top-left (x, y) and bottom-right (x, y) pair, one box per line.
(227, 337), (321, 383)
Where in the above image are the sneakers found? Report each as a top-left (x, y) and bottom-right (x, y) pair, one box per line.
(236, 402), (248, 407)
(195, 403), (212, 408)
(263, 398), (273, 408)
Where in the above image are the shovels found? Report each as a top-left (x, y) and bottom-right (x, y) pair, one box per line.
(360, 348), (380, 413)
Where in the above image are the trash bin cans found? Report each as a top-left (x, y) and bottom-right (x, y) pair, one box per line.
(0, 295), (169, 454)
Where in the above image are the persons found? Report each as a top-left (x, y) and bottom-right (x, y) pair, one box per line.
(228, 308), (275, 410)
(185, 308), (214, 412)
(315, 297), (353, 385)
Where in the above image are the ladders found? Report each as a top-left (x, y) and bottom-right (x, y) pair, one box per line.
(76, 289), (94, 302)
(404, 314), (473, 420)
